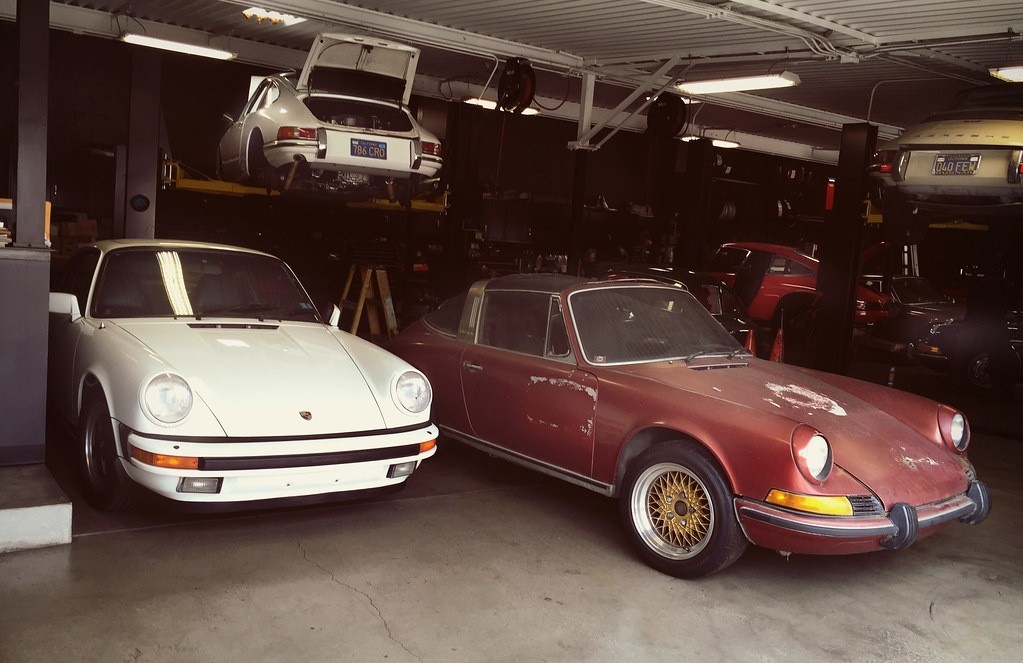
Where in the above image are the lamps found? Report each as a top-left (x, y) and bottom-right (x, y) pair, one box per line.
(461, 80), (542, 115)
(681, 127), (740, 148)
(109, 3), (238, 60)
(673, 46), (802, 94)
(989, 27), (1023, 82)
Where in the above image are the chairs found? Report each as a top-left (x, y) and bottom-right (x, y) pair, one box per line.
(194, 272), (250, 315)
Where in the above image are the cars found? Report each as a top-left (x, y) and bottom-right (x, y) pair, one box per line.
(215, 33), (443, 193)
(864, 83), (1023, 197)
(49, 239), (439, 514)
(379, 273), (992, 579)
(597, 242), (997, 429)
